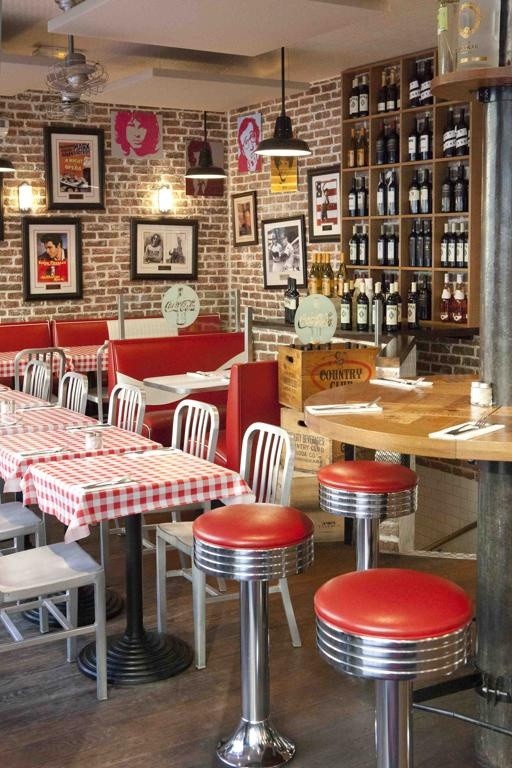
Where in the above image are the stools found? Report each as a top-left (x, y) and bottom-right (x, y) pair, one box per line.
(314, 568), (477, 768)
(317, 459), (419, 571)
(192, 503), (316, 767)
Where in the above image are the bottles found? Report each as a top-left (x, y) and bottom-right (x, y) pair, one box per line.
(283, 251), (469, 334)
(85, 430), (103, 451)
(434, 2), (512, 79)
(346, 57), (473, 269)
(470, 380), (495, 407)
(0, 400), (15, 415)
(286, 342), (370, 354)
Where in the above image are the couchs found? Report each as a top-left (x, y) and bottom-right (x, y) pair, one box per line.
(53, 312), (225, 346)
(0, 317), (52, 352)
(107, 331), (246, 443)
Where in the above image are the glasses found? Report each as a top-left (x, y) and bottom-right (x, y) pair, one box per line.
(243, 131), (256, 150)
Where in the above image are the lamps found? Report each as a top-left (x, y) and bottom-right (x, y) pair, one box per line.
(0, 159), (16, 241)
(183, 111), (227, 179)
(157, 184), (172, 213)
(18, 181), (32, 214)
(253, 46), (312, 158)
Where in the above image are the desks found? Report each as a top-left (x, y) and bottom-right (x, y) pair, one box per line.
(304, 374), (512, 462)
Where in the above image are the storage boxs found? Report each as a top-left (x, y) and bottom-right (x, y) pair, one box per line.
(271, 341), (378, 545)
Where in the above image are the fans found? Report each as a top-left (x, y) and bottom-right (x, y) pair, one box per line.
(47, 95), (93, 119)
(43, 0), (110, 93)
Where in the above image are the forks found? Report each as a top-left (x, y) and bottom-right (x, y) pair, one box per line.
(446, 411), (489, 434)
(377, 375), (427, 387)
(313, 395), (382, 411)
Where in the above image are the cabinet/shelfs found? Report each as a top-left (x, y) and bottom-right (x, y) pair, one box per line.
(337, 45), (482, 330)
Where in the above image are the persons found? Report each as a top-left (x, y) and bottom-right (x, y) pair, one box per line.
(239, 209), (251, 235)
(271, 157), (295, 189)
(278, 232), (294, 270)
(236, 117), (262, 171)
(184, 141), (224, 196)
(269, 233), (283, 272)
(144, 234), (162, 263)
(321, 182), (329, 220)
(113, 111), (162, 160)
(40, 234), (68, 262)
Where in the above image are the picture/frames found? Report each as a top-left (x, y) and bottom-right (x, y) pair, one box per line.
(230, 190), (258, 247)
(129, 216), (198, 281)
(306, 164), (340, 243)
(22, 216), (83, 301)
(42, 124), (107, 211)
(260, 214), (307, 290)
